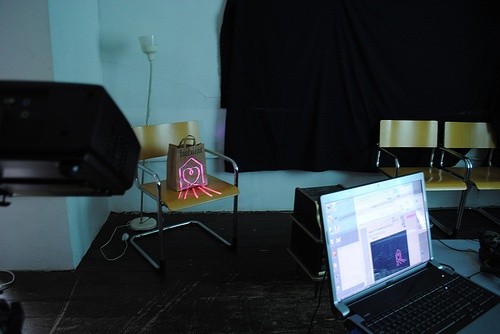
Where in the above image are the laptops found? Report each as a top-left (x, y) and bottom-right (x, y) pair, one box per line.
(317, 171), (500, 334)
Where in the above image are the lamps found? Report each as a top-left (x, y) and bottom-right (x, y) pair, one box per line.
(130, 36), (159, 231)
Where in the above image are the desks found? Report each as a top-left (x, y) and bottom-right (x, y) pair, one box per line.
(50, 211), (500, 334)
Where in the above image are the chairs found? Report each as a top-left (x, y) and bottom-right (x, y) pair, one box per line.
(376, 119), (469, 238)
(441, 122), (500, 237)
(130, 121), (240, 271)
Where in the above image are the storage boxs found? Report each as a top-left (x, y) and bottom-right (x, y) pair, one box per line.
(287, 184), (347, 283)
